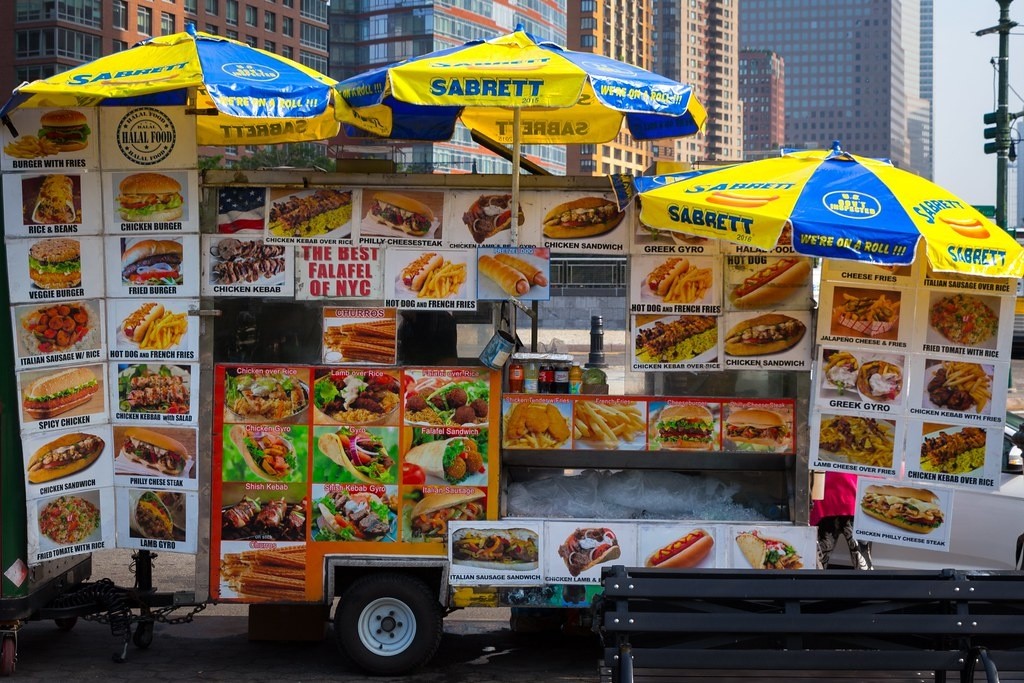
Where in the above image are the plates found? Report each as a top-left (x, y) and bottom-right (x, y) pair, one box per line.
(642, 268), (707, 306)
(35, 494), (101, 551)
(821, 379), (857, 394)
(818, 413), (894, 468)
(269, 190), (352, 239)
(210, 238), (284, 286)
(132, 493), (172, 540)
(118, 334), (174, 351)
(220, 510), (308, 556)
(635, 315), (718, 362)
(922, 426), (983, 477)
(395, 269), (464, 299)
(114, 456), (193, 480)
(119, 365), (190, 414)
(323, 318), (397, 365)
(923, 362), (993, 417)
(357, 210), (438, 240)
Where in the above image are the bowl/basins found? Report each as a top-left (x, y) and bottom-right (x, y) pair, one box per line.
(833, 309), (898, 336)
(404, 376), (490, 429)
(326, 352), (343, 362)
(224, 375), (310, 425)
(313, 373), (402, 425)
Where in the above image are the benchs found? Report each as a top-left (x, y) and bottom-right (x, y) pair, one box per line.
(597, 563), (1024, 682)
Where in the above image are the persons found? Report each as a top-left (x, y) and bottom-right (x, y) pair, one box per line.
(808, 468), (873, 571)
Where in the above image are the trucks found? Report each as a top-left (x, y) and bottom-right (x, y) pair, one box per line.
(190, 177), (1024, 668)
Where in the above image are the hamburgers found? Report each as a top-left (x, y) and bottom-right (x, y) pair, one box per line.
(4, 101), (204, 492)
(639, 399), (793, 456)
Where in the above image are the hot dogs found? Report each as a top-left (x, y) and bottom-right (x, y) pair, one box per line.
(357, 183), (445, 242)
(387, 243), (549, 309)
(640, 520), (713, 572)
(725, 250), (807, 310)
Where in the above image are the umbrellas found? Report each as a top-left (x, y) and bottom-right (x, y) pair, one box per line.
(0, 25), (342, 145)
(333, 24), (711, 252)
(631, 138), (1024, 282)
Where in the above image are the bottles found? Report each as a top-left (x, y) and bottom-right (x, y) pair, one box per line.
(581, 364), (606, 384)
(523, 359), (539, 394)
(509, 358), (523, 393)
(553, 360), (570, 394)
(538, 360), (556, 393)
(569, 361), (584, 394)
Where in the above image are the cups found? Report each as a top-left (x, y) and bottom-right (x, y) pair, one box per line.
(479, 330), (516, 371)
